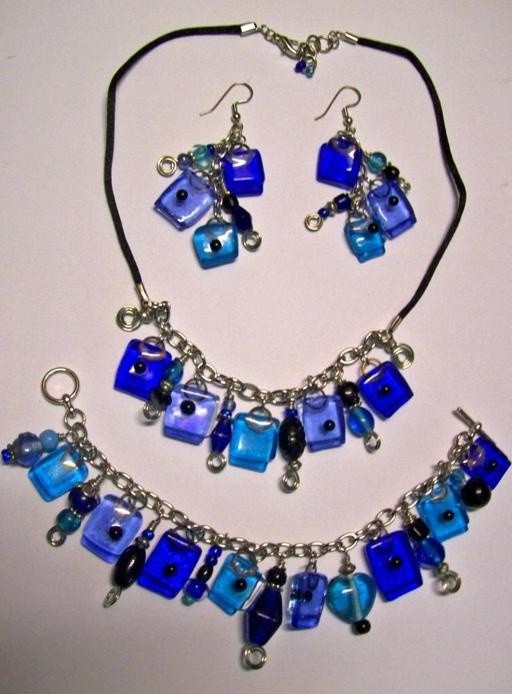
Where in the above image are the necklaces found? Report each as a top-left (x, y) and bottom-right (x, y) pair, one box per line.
(3, 367), (512, 667)
(101, 20), (468, 488)
